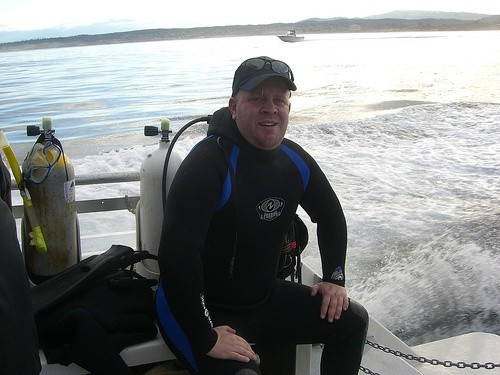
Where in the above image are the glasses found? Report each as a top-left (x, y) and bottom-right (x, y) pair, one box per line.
(241, 57), (294, 82)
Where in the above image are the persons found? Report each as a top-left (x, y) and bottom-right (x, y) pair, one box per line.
(152, 56), (368, 375)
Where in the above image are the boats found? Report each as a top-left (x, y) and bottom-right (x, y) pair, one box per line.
(277, 29), (305, 42)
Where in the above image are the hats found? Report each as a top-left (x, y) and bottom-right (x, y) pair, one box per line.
(232, 56), (297, 96)
